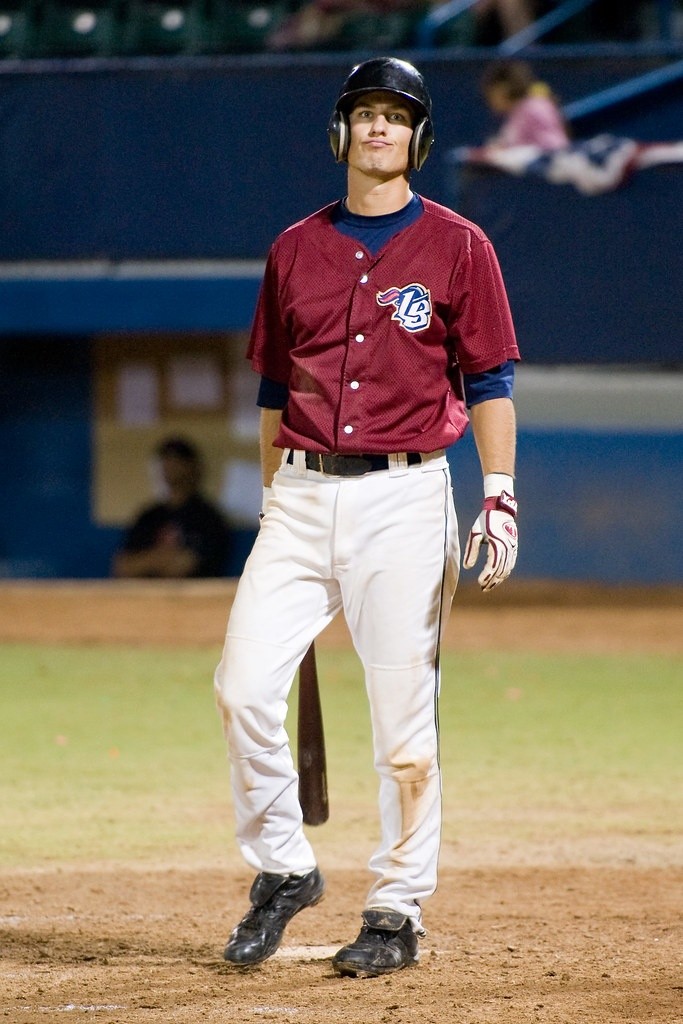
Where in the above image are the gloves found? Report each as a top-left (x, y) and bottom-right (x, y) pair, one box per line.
(462, 474), (519, 592)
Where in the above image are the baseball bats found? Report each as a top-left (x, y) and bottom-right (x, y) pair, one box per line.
(296, 639), (331, 827)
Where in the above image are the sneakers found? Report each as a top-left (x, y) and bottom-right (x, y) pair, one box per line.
(222, 866), (322, 964)
(334, 909), (417, 977)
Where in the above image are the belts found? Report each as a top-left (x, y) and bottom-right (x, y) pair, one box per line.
(287, 448), (423, 476)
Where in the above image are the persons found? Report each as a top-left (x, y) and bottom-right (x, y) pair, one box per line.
(110, 436), (237, 580)
(482, 58), (568, 153)
(212, 56), (521, 980)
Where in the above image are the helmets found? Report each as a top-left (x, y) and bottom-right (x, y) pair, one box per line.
(327, 56), (435, 173)
